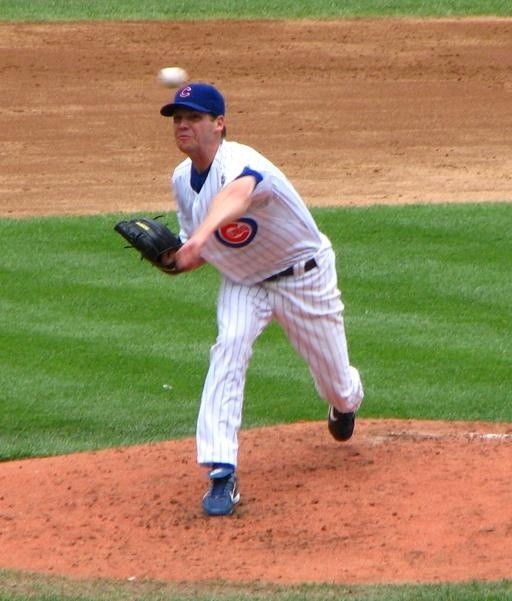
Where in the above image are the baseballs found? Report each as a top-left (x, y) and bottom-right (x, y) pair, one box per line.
(161, 67), (188, 87)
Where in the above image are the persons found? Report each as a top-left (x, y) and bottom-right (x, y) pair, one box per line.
(161, 83), (364, 515)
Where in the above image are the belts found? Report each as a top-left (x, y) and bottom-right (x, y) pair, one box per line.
(264, 258), (317, 281)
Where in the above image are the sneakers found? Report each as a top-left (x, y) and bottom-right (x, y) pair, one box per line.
(328, 404), (355, 442)
(203, 467), (240, 516)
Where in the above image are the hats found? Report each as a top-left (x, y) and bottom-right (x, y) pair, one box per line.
(160, 83), (225, 117)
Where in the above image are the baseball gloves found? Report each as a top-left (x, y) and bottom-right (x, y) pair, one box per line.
(115, 216), (183, 274)
